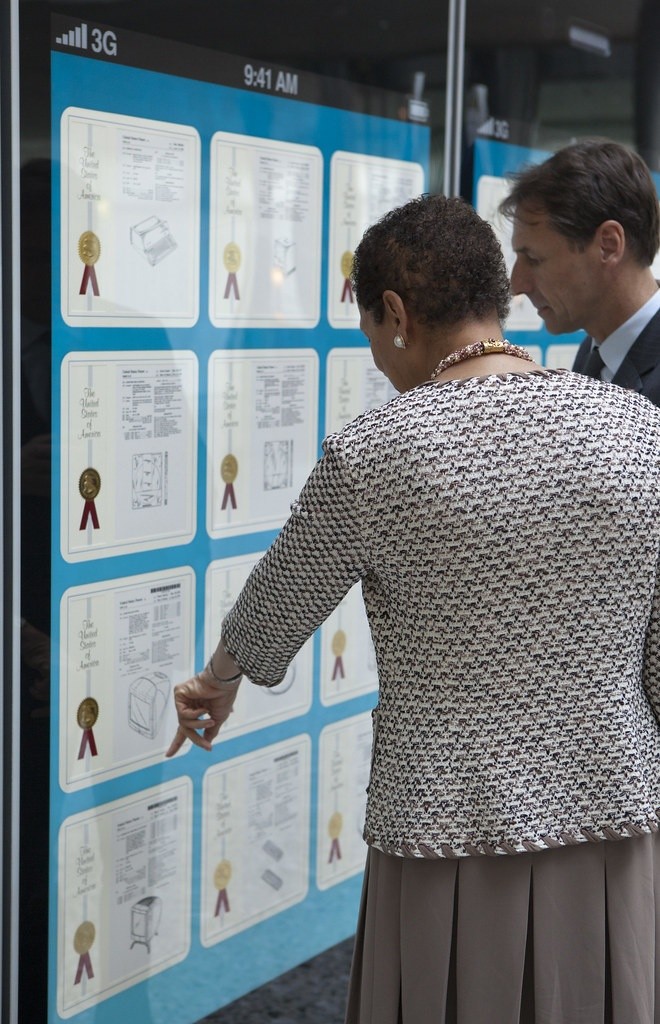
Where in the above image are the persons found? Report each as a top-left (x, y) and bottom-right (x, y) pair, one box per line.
(502, 144), (660, 411)
(167, 194), (660, 1024)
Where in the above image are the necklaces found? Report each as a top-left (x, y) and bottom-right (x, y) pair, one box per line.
(430, 339), (533, 379)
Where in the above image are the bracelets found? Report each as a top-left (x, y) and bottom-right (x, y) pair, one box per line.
(209, 659), (244, 684)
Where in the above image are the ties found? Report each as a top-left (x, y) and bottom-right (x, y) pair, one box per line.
(580, 346), (608, 380)
